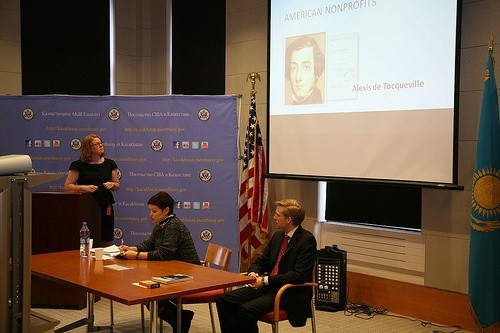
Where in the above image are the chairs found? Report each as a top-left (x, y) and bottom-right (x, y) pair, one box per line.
(238, 261), (318, 333)
(140, 242), (232, 333)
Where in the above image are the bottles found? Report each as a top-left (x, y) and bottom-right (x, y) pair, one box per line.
(80, 222), (90, 258)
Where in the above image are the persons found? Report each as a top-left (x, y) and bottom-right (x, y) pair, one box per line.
(216, 199), (317, 333)
(64, 133), (120, 247)
(285, 37), (325, 105)
(118, 191), (201, 333)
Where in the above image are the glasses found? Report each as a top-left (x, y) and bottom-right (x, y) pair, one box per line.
(90, 141), (104, 146)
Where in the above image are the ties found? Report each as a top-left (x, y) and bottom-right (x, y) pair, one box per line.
(269, 235), (291, 276)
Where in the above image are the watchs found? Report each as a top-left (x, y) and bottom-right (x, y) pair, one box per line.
(136, 251), (140, 260)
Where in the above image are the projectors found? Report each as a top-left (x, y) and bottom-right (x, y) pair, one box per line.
(0, 154), (33, 175)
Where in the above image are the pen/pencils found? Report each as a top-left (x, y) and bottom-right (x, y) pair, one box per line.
(121, 238), (124, 253)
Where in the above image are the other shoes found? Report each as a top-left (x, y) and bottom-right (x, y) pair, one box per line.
(172, 310), (194, 333)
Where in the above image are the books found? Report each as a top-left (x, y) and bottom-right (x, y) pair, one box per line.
(88, 245), (125, 256)
(152, 274), (193, 284)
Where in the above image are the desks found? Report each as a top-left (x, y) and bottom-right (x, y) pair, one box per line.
(30, 247), (257, 333)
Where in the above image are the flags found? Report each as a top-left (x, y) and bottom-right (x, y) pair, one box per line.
(238, 89), (269, 263)
(468, 51), (500, 328)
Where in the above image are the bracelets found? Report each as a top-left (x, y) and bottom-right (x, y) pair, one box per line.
(262, 277), (266, 286)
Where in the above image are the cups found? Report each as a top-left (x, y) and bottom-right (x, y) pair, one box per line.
(95, 248), (103, 261)
(90, 239), (93, 249)
